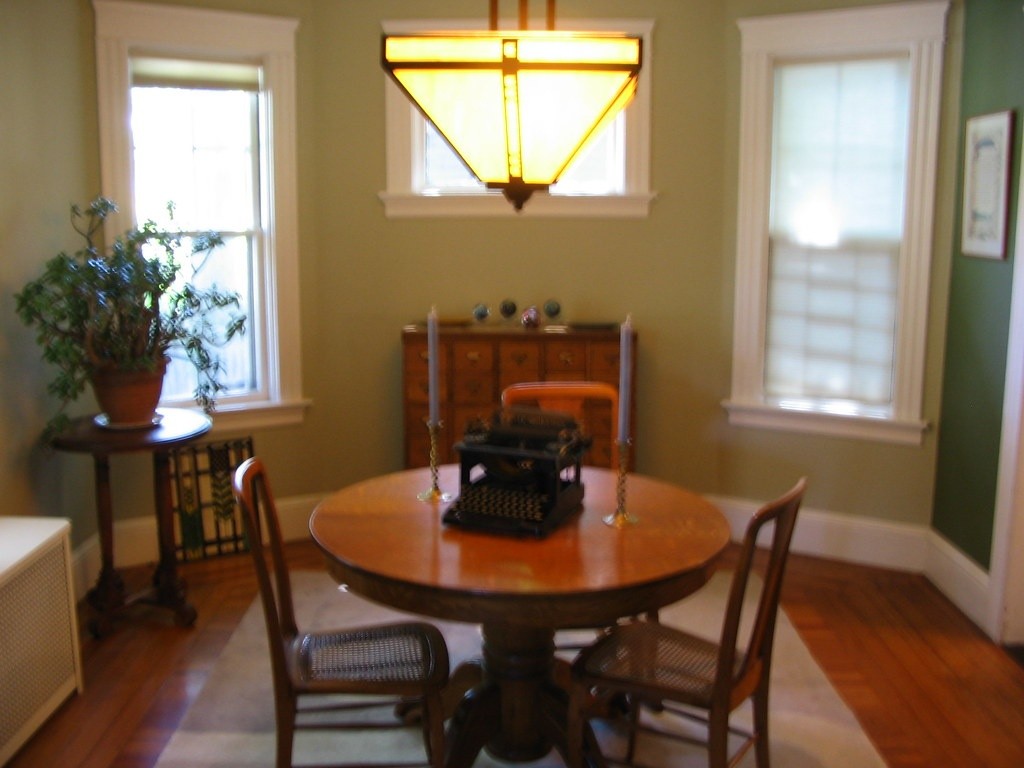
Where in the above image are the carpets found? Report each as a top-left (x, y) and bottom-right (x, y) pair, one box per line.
(152, 564), (888, 768)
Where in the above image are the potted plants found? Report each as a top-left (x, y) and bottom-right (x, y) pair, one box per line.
(12, 192), (247, 449)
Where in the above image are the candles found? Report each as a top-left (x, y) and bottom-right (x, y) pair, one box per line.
(617, 313), (632, 440)
(427, 306), (441, 423)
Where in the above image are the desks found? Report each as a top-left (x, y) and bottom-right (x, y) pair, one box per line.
(46, 404), (212, 637)
(308, 455), (731, 768)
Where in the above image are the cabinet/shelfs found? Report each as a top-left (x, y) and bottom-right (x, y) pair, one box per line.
(401, 317), (638, 469)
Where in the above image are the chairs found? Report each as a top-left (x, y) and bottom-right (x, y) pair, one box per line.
(234, 455), (451, 767)
(565, 472), (810, 768)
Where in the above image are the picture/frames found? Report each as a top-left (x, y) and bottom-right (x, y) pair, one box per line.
(961, 107), (1017, 262)
